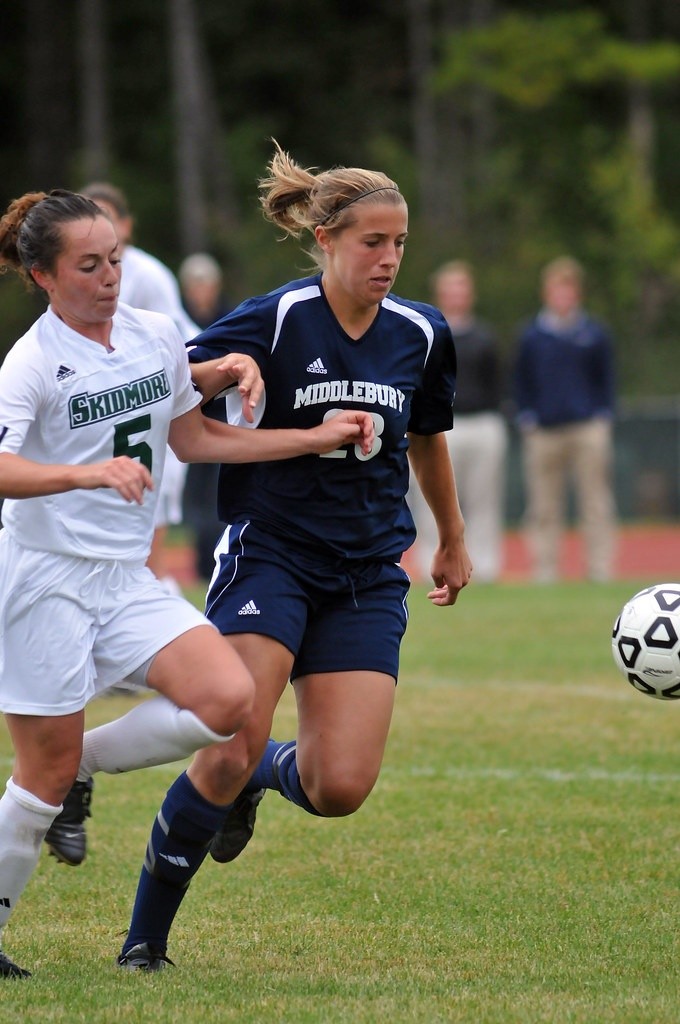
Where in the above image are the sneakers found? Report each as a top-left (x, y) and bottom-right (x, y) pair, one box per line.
(210, 738), (276, 864)
(118, 942), (175, 973)
(44, 776), (93, 866)
(0, 949), (32, 980)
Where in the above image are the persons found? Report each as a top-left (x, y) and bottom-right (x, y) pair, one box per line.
(0, 190), (376, 978)
(175, 254), (234, 583)
(511, 257), (626, 585)
(86, 180), (204, 572)
(411, 260), (514, 584)
(120, 146), (476, 971)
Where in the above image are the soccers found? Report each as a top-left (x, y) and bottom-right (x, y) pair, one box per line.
(611, 583), (680, 701)
